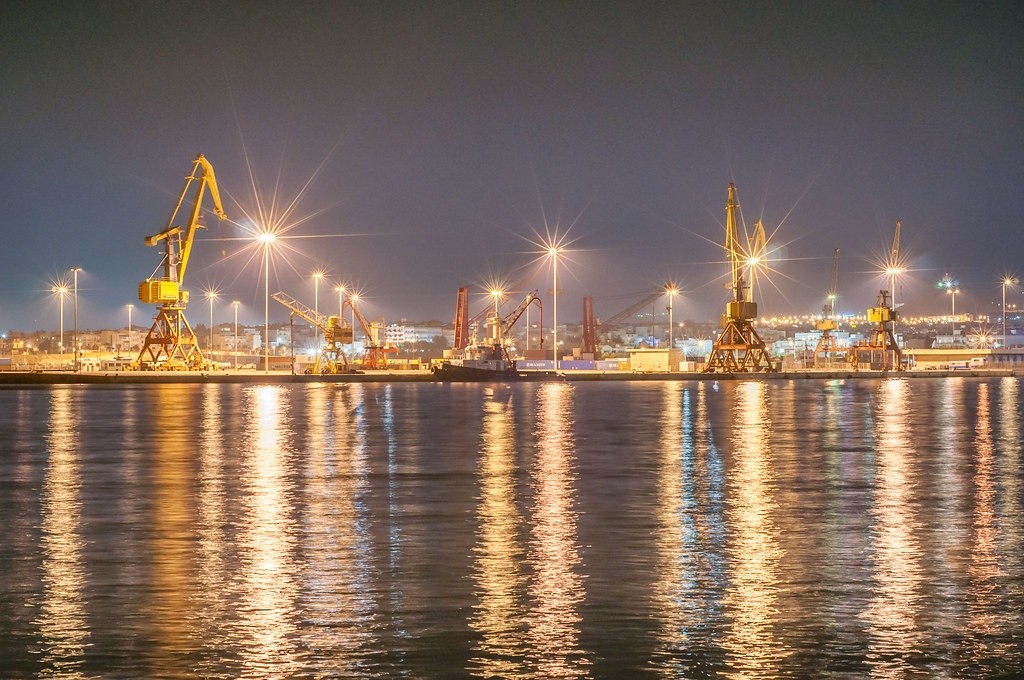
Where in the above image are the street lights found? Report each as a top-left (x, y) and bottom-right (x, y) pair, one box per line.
(260, 233), (278, 372)
(232, 300), (241, 368)
(205, 291), (218, 364)
(71, 267), (82, 368)
(666, 289), (677, 348)
(550, 248), (557, 371)
(312, 273), (323, 363)
(349, 294), (358, 365)
(887, 268), (900, 370)
(1002, 280), (1011, 348)
(748, 258), (758, 371)
(336, 287), (345, 327)
(126, 304), (134, 354)
(948, 289), (959, 335)
(492, 291), (501, 315)
(58, 287), (67, 363)
(828, 295), (835, 316)
(526, 296), (531, 350)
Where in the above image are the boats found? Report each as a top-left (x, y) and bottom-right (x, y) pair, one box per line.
(430, 341), (519, 381)
